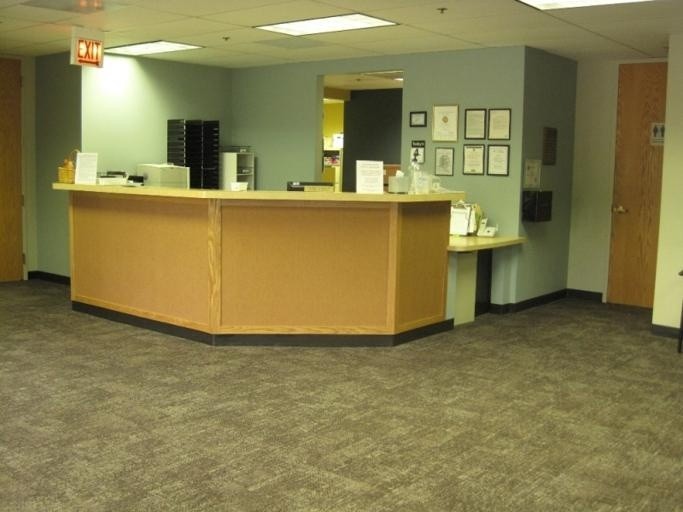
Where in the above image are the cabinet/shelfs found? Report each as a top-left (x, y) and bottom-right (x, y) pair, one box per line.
(165, 118), (220, 188)
(522, 190), (553, 224)
(218, 149), (256, 190)
(136, 163), (190, 189)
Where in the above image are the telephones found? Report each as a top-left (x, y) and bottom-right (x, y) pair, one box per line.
(476, 218), (498, 237)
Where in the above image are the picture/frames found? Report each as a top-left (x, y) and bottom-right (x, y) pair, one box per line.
(486, 104), (512, 142)
(408, 111), (428, 127)
(462, 108), (486, 140)
(461, 143), (485, 178)
(433, 146), (455, 178)
(430, 103), (459, 142)
(485, 144), (512, 178)
